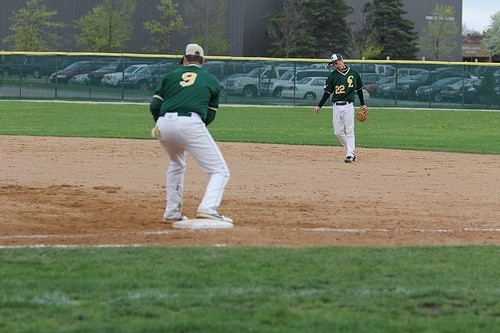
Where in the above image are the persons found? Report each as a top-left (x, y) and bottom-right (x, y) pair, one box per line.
(149, 44), (236, 223)
(314, 53), (368, 163)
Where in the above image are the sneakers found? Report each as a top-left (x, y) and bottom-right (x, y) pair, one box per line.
(344, 156), (356, 163)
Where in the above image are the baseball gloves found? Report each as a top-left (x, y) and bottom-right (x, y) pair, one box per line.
(355, 105), (369, 123)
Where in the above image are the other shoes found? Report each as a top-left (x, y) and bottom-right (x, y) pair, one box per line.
(196, 213), (235, 223)
(163, 215), (189, 223)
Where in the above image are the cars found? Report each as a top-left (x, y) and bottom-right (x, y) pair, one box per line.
(50, 62), (499, 104)
(0, 56), (43, 79)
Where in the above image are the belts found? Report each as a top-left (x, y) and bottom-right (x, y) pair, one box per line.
(334, 102), (351, 106)
(159, 112), (193, 117)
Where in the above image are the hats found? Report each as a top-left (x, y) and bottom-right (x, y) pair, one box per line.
(328, 54), (343, 65)
(179, 44), (203, 65)
